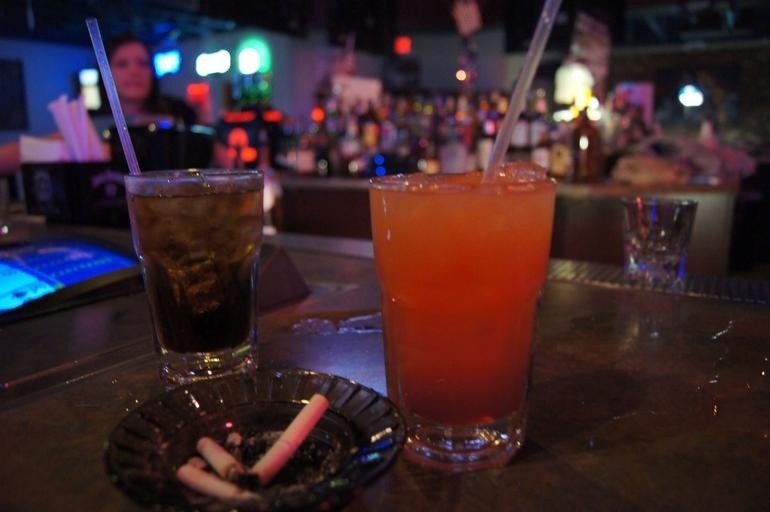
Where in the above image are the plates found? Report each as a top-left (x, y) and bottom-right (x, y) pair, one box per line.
(103, 369), (408, 510)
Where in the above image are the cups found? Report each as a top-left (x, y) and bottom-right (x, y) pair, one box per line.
(126, 167), (277, 386)
(367, 171), (561, 473)
(622, 193), (697, 293)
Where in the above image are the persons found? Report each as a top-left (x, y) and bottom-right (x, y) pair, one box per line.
(53, 30), (210, 223)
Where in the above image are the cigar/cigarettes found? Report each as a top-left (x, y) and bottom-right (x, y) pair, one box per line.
(197, 434), (243, 484)
(175, 461), (261, 508)
(248, 394), (329, 492)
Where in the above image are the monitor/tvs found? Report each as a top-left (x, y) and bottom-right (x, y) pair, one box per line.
(0, 237), (145, 323)
